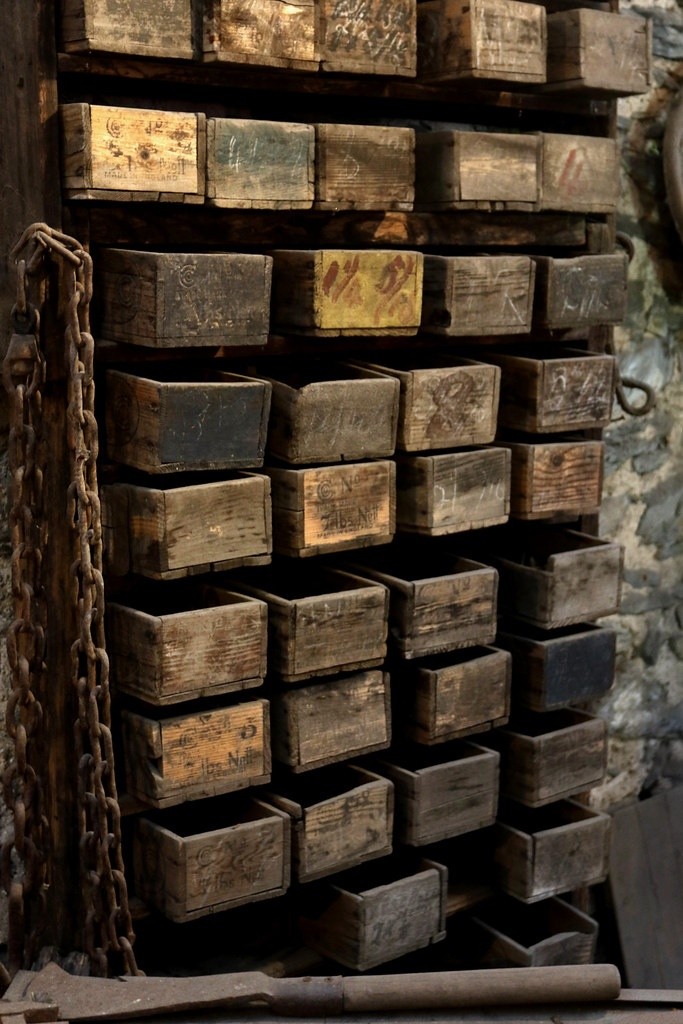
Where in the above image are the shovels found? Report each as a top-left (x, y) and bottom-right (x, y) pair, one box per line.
(4, 959), (632, 1020)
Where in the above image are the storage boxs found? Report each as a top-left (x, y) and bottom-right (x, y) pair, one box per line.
(56, 1), (658, 970)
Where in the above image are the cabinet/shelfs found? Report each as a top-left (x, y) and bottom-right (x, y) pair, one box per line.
(2, 1), (617, 978)
